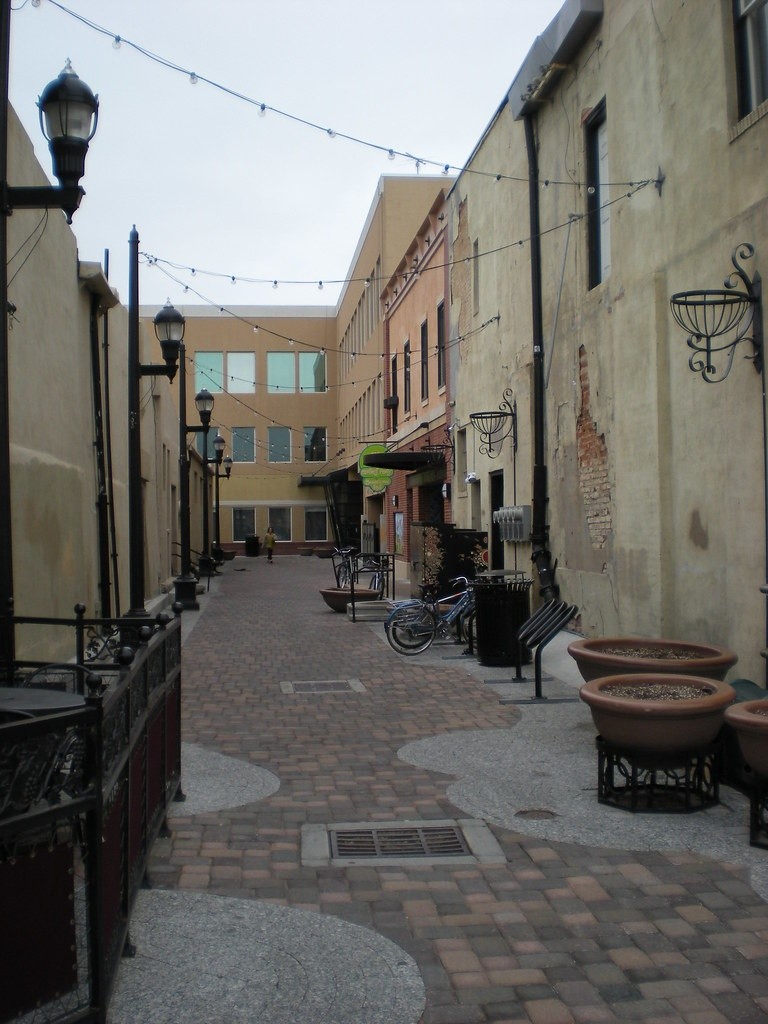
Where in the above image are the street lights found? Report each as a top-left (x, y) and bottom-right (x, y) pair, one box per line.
(121, 298), (183, 673)
(214, 455), (232, 566)
(3, 63), (95, 682)
(199, 436), (226, 578)
(175, 389), (216, 608)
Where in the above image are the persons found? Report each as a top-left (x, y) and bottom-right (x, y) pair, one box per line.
(262, 527), (277, 564)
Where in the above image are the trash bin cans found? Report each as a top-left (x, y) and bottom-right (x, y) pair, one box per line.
(245, 534), (260, 558)
(467, 569), (534, 665)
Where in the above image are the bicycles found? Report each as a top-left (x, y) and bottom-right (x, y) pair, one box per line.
(332, 546), (359, 588)
(383, 574), (478, 656)
(365, 558), (386, 599)
